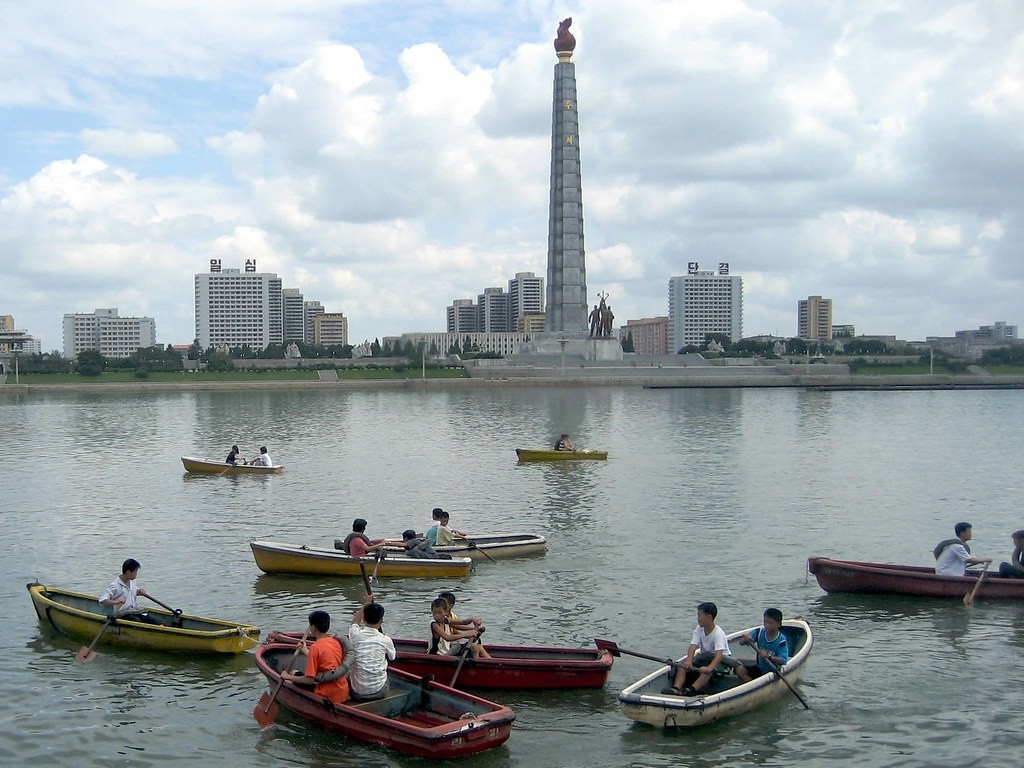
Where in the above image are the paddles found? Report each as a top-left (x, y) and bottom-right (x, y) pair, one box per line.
(461, 537), (498, 564)
(76, 595), (127, 662)
(253, 625), (311, 727)
(369, 546), (384, 583)
(139, 589), (175, 613)
(221, 459), (243, 474)
(415, 532), (467, 538)
(963, 561), (990, 607)
(449, 623), (479, 688)
(747, 641), (810, 711)
(358, 560), (396, 661)
(593, 637), (712, 676)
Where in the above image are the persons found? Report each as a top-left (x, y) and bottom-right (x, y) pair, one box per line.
(250, 446), (272, 467)
(588, 304), (614, 338)
(377, 530), (439, 559)
(225, 445), (247, 466)
(348, 594), (396, 704)
(661, 601), (734, 696)
(98, 558), (165, 627)
(425, 508), (467, 546)
(426, 592), (493, 658)
(343, 518), (386, 558)
(934, 522), (993, 577)
(553, 433), (576, 451)
(734, 608), (789, 685)
(999, 530), (1024, 580)
(280, 611), (350, 706)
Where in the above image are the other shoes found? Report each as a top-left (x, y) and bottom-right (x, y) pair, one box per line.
(661, 687), (681, 695)
(682, 688), (693, 696)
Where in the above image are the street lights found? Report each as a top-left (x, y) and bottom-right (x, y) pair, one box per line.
(10, 347), (23, 384)
(419, 340), (428, 378)
(926, 339), (938, 374)
(803, 339), (814, 376)
(558, 340), (569, 375)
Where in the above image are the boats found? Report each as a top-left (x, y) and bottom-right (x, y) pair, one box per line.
(254, 643), (516, 762)
(618, 615), (814, 731)
(250, 541), (472, 578)
(27, 582), (261, 658)
(181, 456), (283, 474)
(264, 630), (614, 691)
(383, 532), (547, 562)
(808, 555), (1024, 600)
(515, 448), (609, 462)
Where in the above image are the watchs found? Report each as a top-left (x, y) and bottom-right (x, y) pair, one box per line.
(388, 541), (391, 545)
(767, 653), (772, 661)
